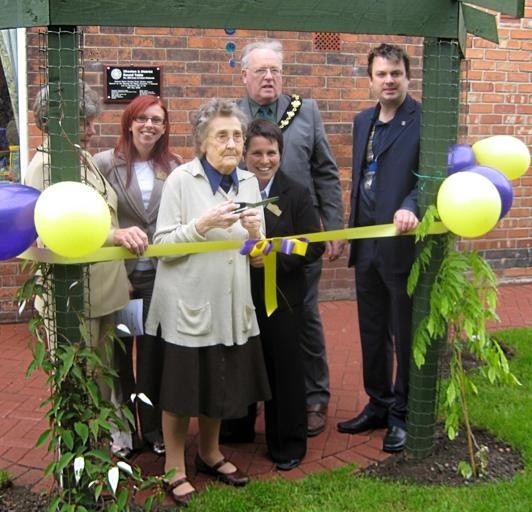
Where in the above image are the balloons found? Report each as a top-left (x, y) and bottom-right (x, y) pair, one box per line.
(0, 184), (41, 260)
(0, 181), (112, 262)
(436, 134), (531, 238)
(34, 181), (112, 262)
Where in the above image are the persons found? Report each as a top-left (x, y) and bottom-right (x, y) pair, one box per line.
(337, 44), (422, 452)
(146, 98), (267, 507)
(231, 40), (345, 435)
(221, 120), (325, 471)
(25, 81), (149, 443)
(95, 94), (184, 459)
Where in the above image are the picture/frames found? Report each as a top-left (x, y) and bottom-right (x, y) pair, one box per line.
(101, 62), (165, 105)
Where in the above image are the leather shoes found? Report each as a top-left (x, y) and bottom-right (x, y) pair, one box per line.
(383, 426), (407, 451)
(307, 403), (327, 436)
(338, 413), (386, 433)
(114, 441), (249, 507)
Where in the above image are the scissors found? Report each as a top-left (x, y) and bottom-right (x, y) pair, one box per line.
(233, 196), (280, 217)
